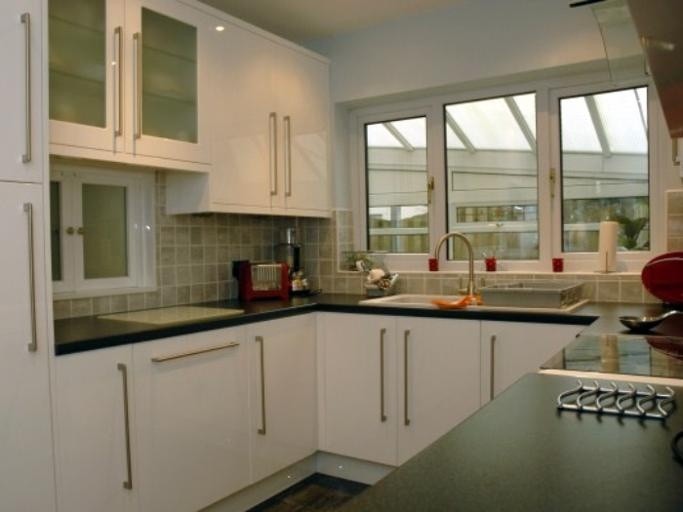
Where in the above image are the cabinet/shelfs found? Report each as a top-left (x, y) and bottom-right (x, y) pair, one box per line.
(50, 345), (140, 512)
(211, 16), (332, 213)
(1, 181), (57, 512)
(481, 319), (587, 410)
(246, 312), (318, 484)
(133, 326), (250, 512)
(1, 1), (44, 184)
(319, 312), (481, 469)
(48, 1), (211, 168)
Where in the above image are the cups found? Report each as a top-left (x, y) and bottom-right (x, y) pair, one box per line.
(486, 258), (497, 272)
(552, 258), (563, 272)
(429, 258), (439, 271)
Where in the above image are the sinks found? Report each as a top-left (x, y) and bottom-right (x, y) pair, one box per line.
(359, 293), (467, 310)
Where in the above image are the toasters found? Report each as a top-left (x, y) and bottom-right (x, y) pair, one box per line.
(239, 261), (289, 302)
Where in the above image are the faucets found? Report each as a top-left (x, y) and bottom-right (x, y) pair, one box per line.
(435, 232), (474, 279)
